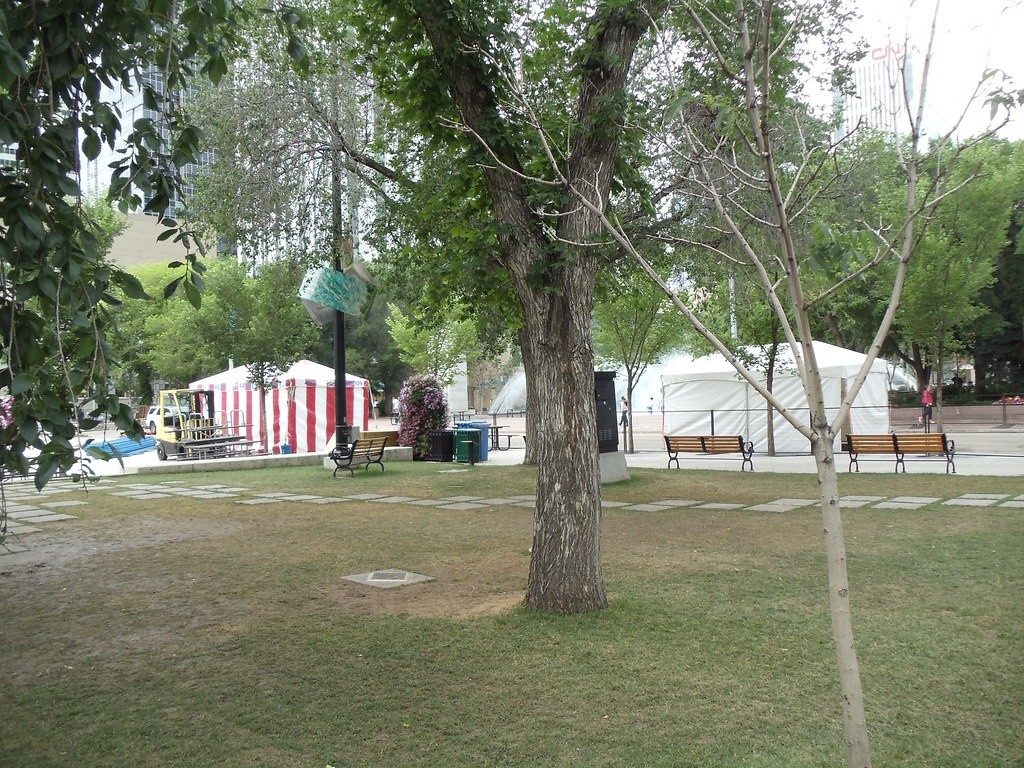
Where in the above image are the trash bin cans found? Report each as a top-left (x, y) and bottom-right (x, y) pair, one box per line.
(429, 430), (454, 462)
(454, 421), (490, 463)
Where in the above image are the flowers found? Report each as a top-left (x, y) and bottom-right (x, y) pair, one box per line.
(398, 373), (451, 457)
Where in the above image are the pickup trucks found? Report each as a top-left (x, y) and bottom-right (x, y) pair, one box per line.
(146, 405), (190, 434)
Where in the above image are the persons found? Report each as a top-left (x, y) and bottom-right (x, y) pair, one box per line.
(922, 384), (935, 424)
(373, 399), (379, 419)
(619, 396), (628, 426)
(951, 374), (963, 386)
(647, 397), (654, 414)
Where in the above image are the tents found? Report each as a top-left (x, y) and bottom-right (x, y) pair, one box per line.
(660, 340), (917, 452)
(189, 359), (369, 455)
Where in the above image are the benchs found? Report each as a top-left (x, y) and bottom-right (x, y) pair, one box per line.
(329, 436), (389, 477)
(455, 414), (474, 421)
(845, 433), (956, 475)
(489, 434), (519, 436)
(664, 435), (755, 471)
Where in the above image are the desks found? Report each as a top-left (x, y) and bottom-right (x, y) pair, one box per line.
(488, 425), (510, 451)
(457, 410), (470, 420)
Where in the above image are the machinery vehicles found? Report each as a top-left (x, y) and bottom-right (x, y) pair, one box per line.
(155, 390), (229, 460)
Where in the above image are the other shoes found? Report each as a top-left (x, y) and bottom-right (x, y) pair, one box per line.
(929, 420), (935, 423)
(918, 416), (922, 422)
(626, 424), (628, 426)
(619, 423), (621, 426)
